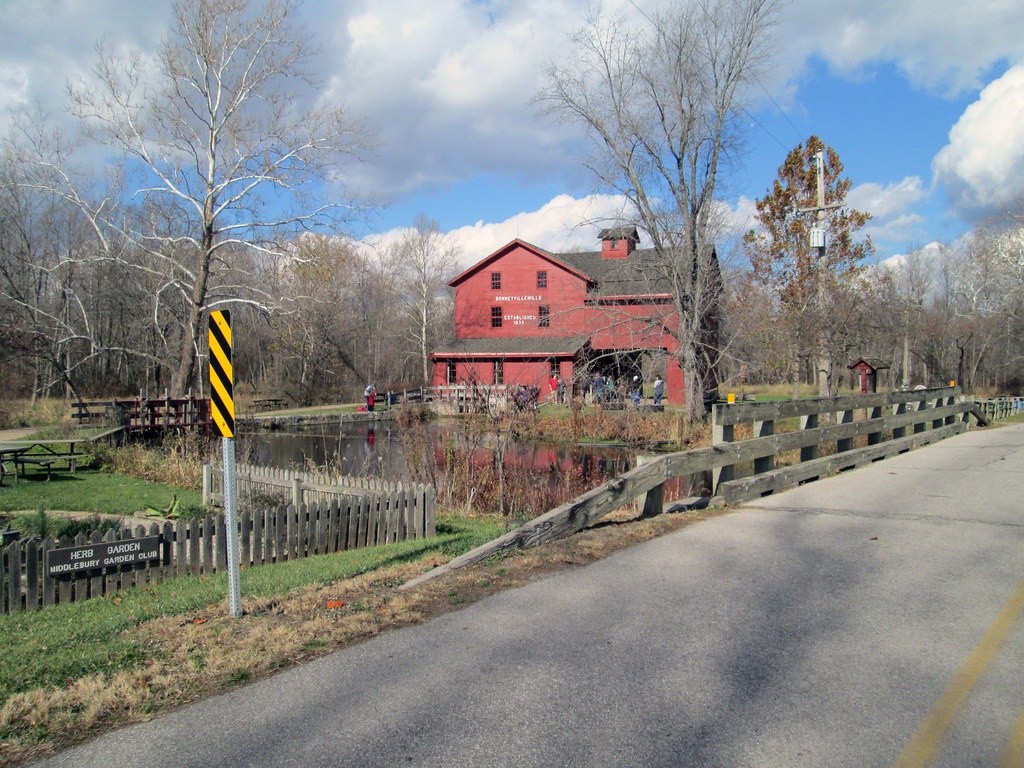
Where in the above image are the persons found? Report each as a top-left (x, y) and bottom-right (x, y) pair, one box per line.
(364, 381), (377, 411)
(653, 375), (665, 405)
(520, 384), (528, 404)
(592, 371), (626, 404)
(366, 421), (375, 453)
(549, 372), (567, 405)
(625, 375), (644, 405)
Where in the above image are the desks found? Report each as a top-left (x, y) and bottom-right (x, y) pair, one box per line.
(0, 439), (87, 472)
(0, 445), (31, 485)
(253, 399), (283, 412)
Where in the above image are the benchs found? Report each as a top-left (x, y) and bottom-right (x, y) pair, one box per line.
(249, 403), (289, 412)
(0, 452), (89, 485)
